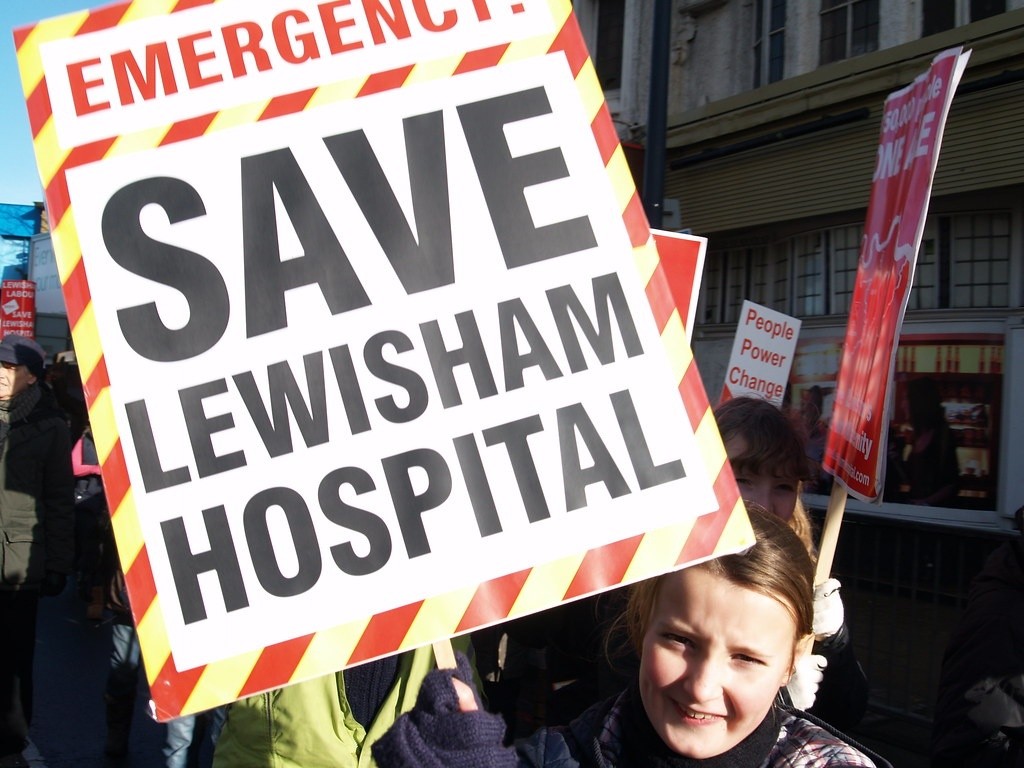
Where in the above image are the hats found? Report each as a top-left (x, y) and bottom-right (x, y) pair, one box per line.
(0, 334), (47, 375)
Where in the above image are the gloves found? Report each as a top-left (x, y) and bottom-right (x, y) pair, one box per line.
(787, 652), (827, 711)
(811, 578), (844, 637)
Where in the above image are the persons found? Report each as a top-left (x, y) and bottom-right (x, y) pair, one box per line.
(0, 332), (224, 768)
(803, 384), (835, 496)
(376, 506), (879, 768)
(212, 632), (490, 768)
(899, 378), (961, 506)
(714, 395), (850, 710)
(927, 506), (1024, 768)
